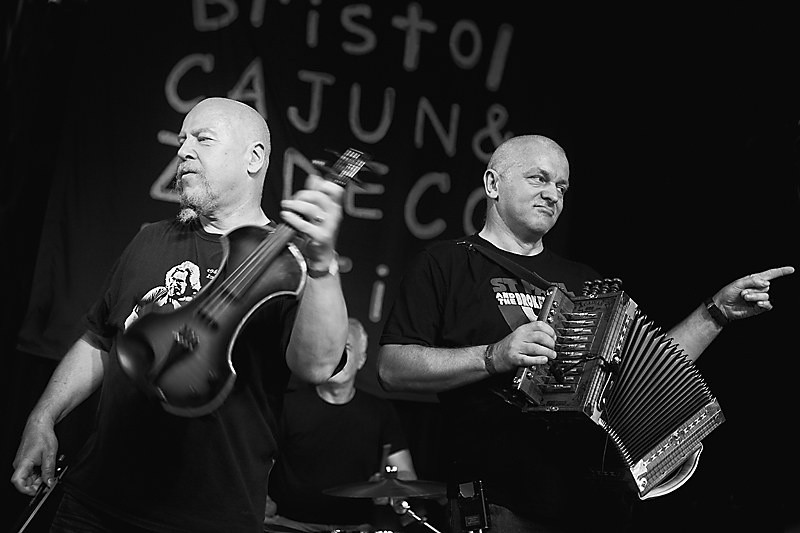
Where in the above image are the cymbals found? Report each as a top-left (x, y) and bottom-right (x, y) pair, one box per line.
(318, 475), (450, 498)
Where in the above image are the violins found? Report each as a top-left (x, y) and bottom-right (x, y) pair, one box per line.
(117, 144), (372, 416)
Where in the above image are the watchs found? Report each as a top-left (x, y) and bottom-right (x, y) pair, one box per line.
(306, 251), (342, 279)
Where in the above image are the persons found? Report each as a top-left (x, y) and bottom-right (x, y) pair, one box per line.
(11, 96), (350, 533)
(266, 318), (446, 533)
(373, 135), (797, 533)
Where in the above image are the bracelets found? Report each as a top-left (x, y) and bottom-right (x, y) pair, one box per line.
(702, 297), (730, 326)
(485, 344), (500, 374)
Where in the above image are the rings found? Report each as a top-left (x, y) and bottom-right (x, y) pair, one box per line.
(312, 213), (325, 226)
